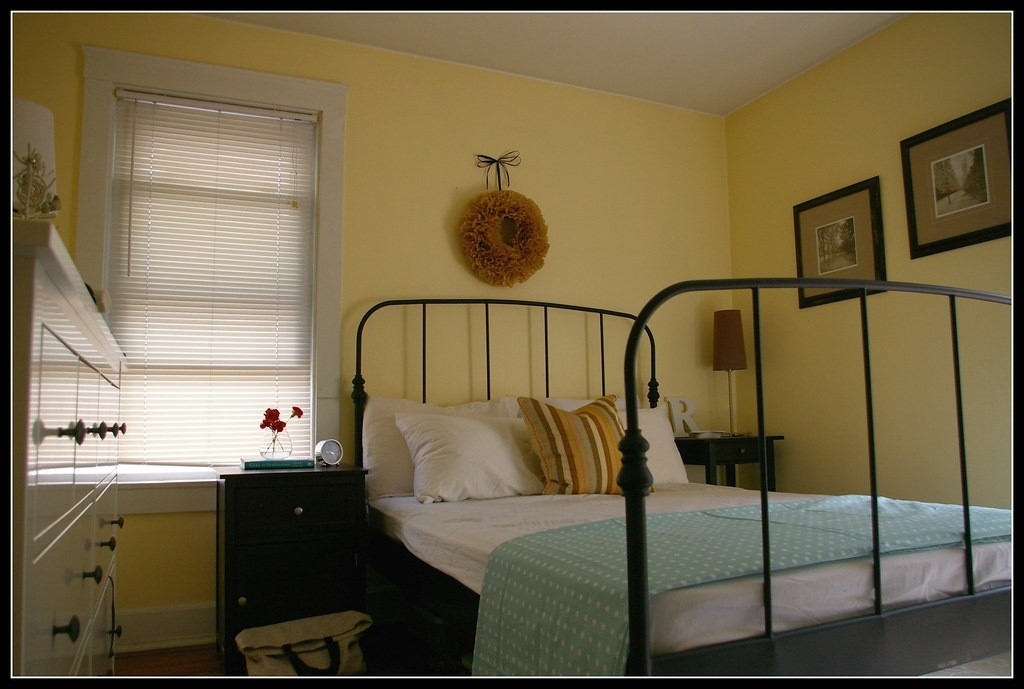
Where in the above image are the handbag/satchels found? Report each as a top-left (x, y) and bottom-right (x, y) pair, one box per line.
(236, 610), (373, 676)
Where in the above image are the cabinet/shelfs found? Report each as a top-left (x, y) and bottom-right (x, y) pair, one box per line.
(11, 218), (134, 674)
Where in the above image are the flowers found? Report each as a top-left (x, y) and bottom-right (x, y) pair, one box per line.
(260, 406), (303, 432)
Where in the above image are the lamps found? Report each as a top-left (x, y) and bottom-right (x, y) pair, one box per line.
(714, 310), (749, 435)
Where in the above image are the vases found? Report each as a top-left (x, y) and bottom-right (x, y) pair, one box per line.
(259, 428), (292, 460)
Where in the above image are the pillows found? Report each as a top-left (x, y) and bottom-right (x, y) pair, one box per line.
(394, 411), (546, 504)
(515, 395), (655, 495)
(362, 395), (521, 500)
(540, 398), (691, 484)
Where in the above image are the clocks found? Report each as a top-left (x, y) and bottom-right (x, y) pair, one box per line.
(314, 439), (343, 467)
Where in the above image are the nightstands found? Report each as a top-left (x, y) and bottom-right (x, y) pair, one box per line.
(217, 467), (369, 673)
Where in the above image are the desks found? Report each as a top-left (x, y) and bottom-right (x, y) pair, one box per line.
(676, 435), (785, 492)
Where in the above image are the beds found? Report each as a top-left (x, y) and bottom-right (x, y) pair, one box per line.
(350, 277), (1012, 678)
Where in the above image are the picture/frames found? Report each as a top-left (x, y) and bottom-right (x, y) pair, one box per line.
(899, 98), (1012, 261)
(793, 175), (887, 310)
